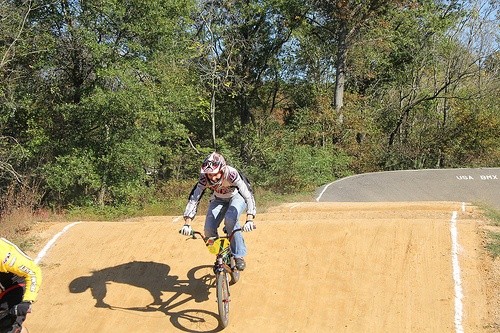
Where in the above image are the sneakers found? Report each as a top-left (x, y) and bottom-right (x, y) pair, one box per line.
(235, 258), (246, 271)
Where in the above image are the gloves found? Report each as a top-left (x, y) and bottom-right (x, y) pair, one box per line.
(181, 225), (191, 236)
(241, 220), (254, 232)
(10, 302), (30, 316)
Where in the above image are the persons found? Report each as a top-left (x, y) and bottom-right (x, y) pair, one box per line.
(180, 154), (256, 274)
(0, 237), (42, 333)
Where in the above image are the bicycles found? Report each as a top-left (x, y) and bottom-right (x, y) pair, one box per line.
(179, 226), (257, 328)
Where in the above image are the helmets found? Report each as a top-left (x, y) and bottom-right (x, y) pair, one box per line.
(201, 152), (226, 189)
